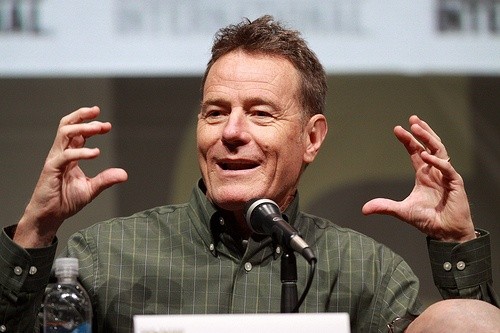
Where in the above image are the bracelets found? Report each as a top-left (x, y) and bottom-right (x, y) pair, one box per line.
(474, 230), (481, 238)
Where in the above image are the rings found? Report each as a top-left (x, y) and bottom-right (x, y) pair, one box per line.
(446, 156), (451, 162)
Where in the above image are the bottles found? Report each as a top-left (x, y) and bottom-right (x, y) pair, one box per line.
(41, 258), (93, 333)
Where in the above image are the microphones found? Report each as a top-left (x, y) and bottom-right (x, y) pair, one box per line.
(244, 198), (317, 264)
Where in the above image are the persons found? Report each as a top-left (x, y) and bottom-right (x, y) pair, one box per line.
(0, 11), (500, 333)
